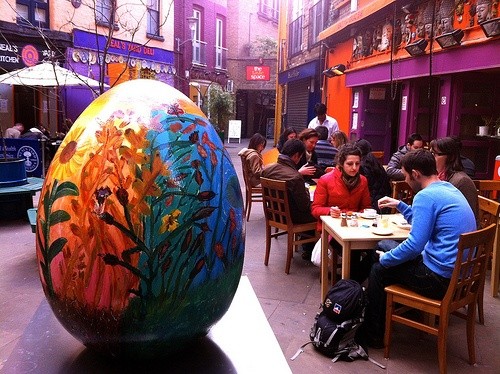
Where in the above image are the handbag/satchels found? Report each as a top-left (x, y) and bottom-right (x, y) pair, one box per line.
(310, 238), (333, 272)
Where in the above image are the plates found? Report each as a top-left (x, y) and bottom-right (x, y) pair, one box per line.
(360, 213), (379, 218)
(372, 227), (393, 235)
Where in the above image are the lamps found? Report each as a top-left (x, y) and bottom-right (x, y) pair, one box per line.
(480, 17), (500, 38)
(435, 29), (464, 49)
(405, 39), (429, 56)
(322, 64), (346, 78)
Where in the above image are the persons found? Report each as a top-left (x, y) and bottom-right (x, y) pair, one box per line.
(3, 117), (74, 156)
(310, 144), (372, 284)
(361, 148), (477, 348)
(262, 138), (315, 263)
(430, 137), (481, 230)
(240, 101), (477, 200)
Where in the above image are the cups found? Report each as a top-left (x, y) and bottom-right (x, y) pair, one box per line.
(363, 209), (376, 217)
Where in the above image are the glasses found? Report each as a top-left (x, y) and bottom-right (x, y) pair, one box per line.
(431, 152), (446, 156)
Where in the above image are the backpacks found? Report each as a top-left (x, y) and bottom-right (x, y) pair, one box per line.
(290, 280), (386, 369)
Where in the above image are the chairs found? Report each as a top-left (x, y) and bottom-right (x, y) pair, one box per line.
(259, 177), (321, 274)
(384, 223), (496, 374)
(241, 156), (262, 222)
(391, 180), (413, 213)
(477, 195), (500, 324)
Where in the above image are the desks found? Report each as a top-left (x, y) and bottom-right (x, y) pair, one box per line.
(320, 214), (408, 305)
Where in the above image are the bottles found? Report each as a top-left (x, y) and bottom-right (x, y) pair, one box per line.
(341, 210), (347, 226)
(351, 213), (359, 227)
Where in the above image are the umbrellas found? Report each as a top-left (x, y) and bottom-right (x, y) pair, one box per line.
(0, 62), (110, 178)
